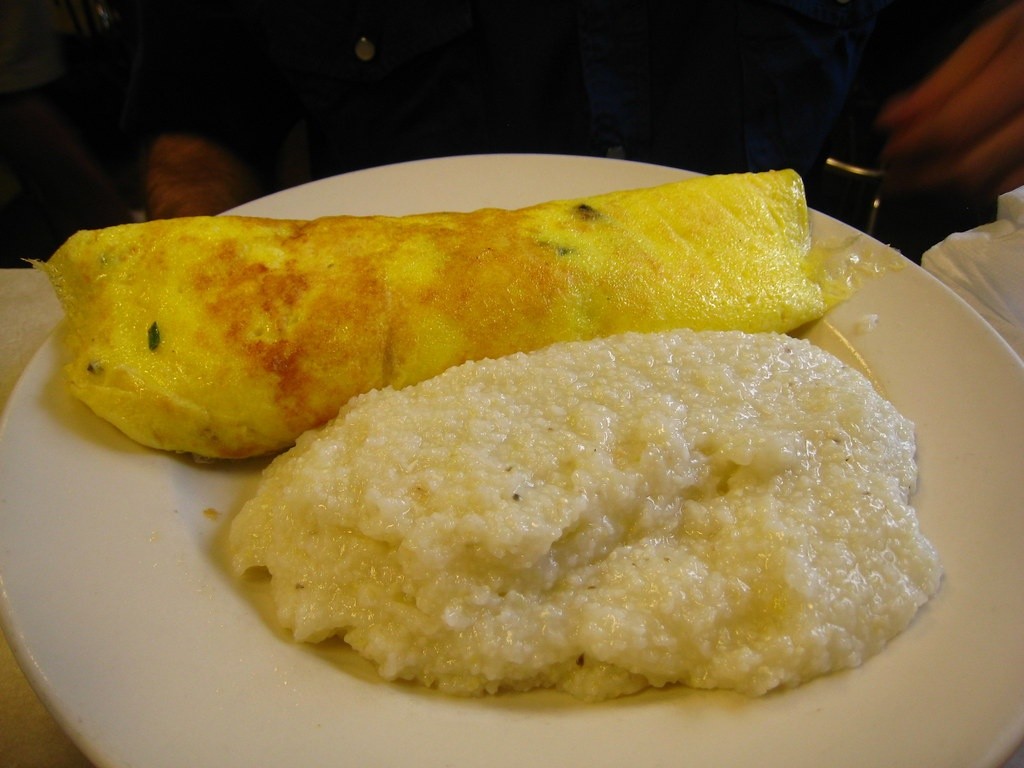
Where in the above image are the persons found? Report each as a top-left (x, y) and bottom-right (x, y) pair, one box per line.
(65, 0), (1024, 266)
(1, 1), (135, 267)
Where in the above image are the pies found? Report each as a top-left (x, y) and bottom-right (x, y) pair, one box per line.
(40, 164), (835, 461)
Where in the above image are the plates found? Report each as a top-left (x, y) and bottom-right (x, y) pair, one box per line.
(1, 155), (1023, 768)
